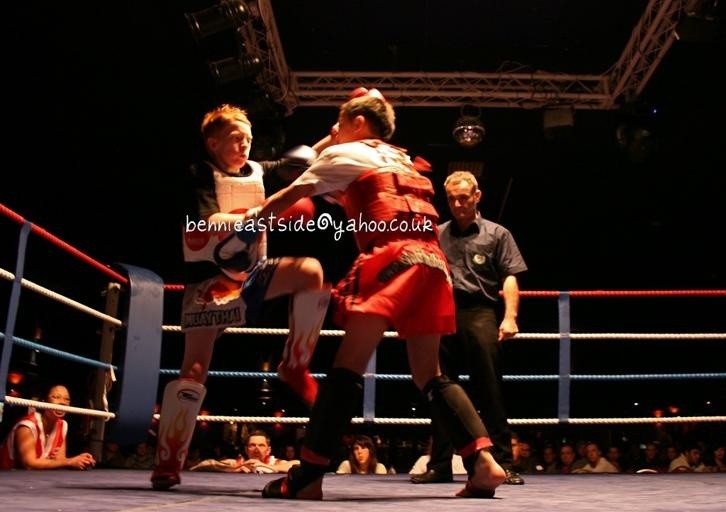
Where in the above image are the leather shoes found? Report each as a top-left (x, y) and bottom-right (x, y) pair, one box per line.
(410, 468), (453, 484)
(503, 468), (524, 485)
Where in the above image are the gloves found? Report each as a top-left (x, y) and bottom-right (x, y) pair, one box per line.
(214, 222), (259, 272)
(276, 197), (314, 226)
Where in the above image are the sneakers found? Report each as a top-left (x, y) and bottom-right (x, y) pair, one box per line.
(279, 364), (317, 403)
(152, 475), (180, 490)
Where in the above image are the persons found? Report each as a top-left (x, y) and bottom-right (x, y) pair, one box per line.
(0, 385), (97, 472)
(150, 85), (386, 494)
(103, 402), (726, 475)
(245, 95), (508, 501)
(408, 169), (529, 486)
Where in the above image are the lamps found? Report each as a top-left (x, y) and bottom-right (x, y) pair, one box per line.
(616, 0), (726, 164)
(181, 0), (266, 92)
